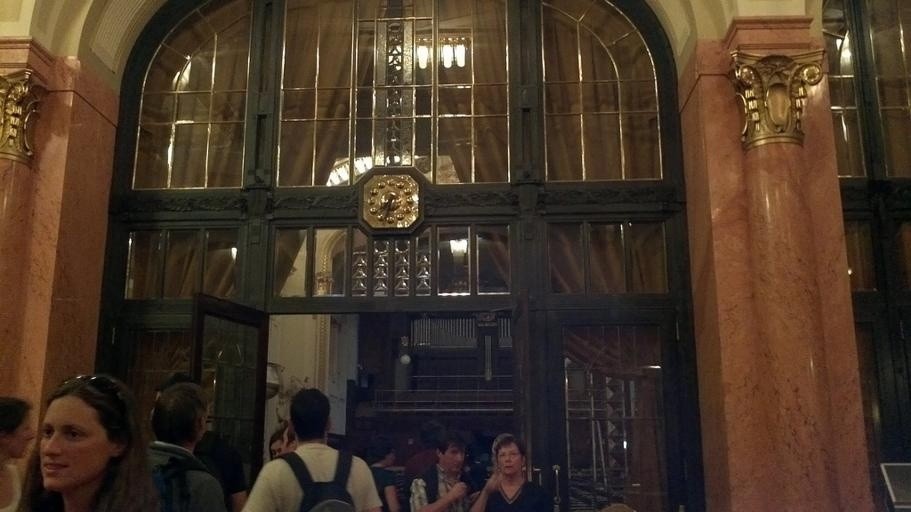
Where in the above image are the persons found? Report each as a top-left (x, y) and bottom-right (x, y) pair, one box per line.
(15, 370), (156, 511)
(152, 380), (248, 511)
(268, 421), (558, 511)
(144, 380), (225, 510)
(242, 385), (385, 511)
(0, 394), (37, 511)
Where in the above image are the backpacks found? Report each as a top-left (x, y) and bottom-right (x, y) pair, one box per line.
(274, 448), (357, 512)
(147, 456), (189, 512)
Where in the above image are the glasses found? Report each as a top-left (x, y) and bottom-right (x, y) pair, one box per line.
(63, 374), (117, 398)
